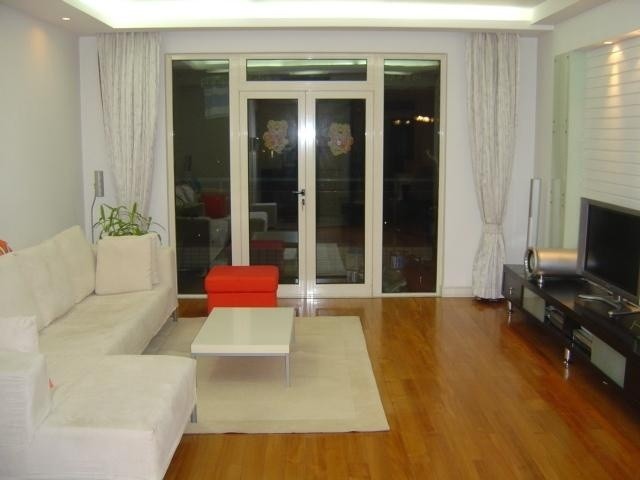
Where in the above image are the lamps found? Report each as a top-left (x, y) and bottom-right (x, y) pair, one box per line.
(91, 170), (104, 243)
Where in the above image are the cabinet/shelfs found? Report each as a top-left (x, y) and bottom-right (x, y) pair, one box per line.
(500, 264), (640, 410)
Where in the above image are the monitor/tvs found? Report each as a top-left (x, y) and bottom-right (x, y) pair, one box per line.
(577, 196), (640, 317)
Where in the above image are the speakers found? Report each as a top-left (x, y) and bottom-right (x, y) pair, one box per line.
(527, 177), (540, 247)
(523, 246), (577, 284)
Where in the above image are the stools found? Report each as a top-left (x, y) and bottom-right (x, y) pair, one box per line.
(230, 208), (269, 238)
(250, 239), (282, 266)
(33, 354), (197, 479)
(204, 264), (280, 313)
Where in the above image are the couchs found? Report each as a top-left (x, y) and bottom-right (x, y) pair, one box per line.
(0, 227), (177, 479)
(174, 182), (231, 272)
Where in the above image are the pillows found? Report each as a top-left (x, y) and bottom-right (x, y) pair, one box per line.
(94, 236), (154, 295)
(179, 192), (227, 218)
(1, 315), (39, 354)
(103, 232), (161, 285)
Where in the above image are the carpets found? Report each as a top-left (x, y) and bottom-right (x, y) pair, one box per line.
(284, 243), (346, 278)
(145, 315), (390, 434)
